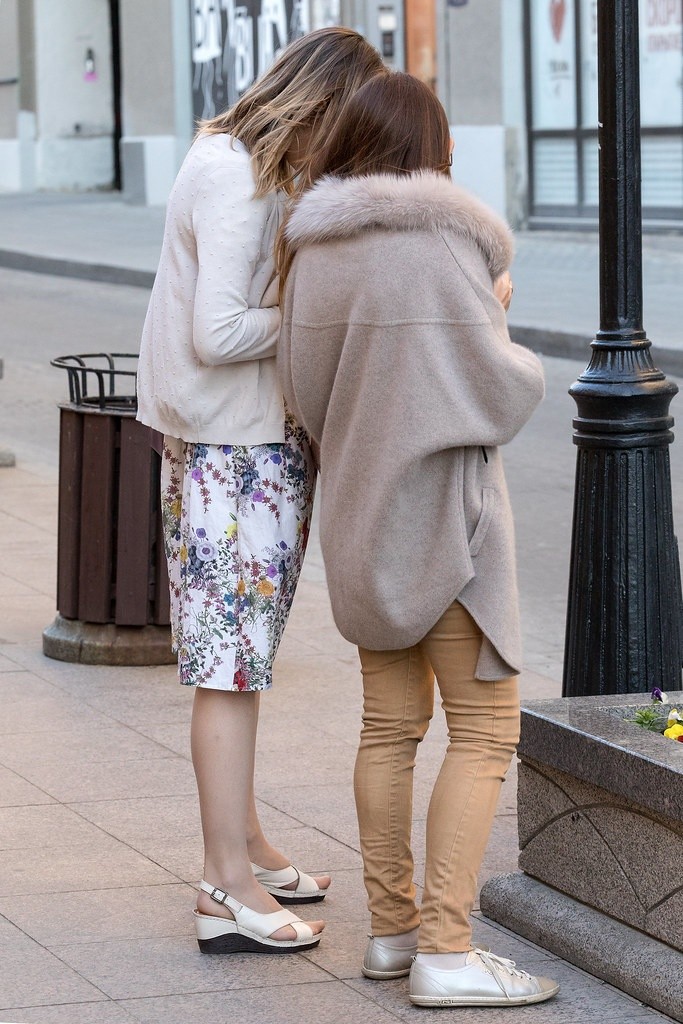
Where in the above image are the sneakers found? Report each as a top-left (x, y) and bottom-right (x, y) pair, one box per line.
(408, 948), (560, 1006)
(360, 933), (418, 979)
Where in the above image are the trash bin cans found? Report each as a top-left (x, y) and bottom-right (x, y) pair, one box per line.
(42, 350), (179, 666)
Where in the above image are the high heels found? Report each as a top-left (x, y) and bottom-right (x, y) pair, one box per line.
(249, 862), (327, 905)
(191, 880), (321, 955)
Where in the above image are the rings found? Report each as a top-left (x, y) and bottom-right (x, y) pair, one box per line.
(508, 280), (513, 295)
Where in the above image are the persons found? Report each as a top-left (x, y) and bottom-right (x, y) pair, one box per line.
(275, 71), (557, 1008)
(135, 26), (395, 954)
